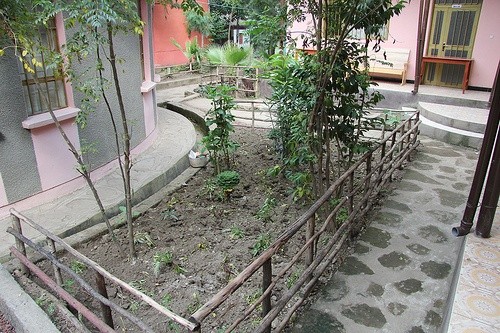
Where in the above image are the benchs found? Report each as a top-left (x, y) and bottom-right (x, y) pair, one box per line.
(345, 45), (411, 85)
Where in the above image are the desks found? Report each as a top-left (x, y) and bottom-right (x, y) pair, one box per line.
(419, 55), (474, 93)
